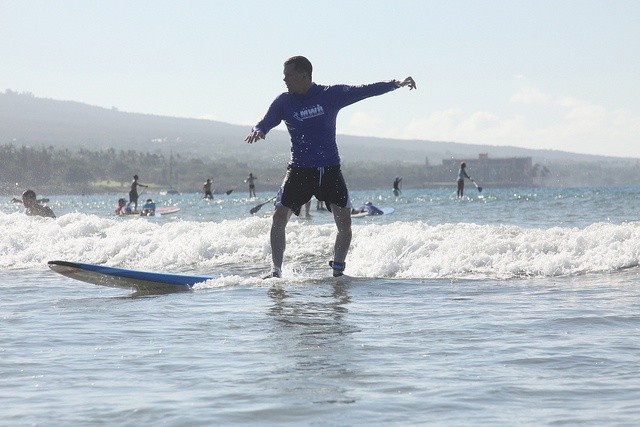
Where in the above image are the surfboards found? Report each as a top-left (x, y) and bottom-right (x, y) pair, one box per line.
(48, 261), (217, 289)
(126, 208), (180, 216)
(351, 208), (394, 217)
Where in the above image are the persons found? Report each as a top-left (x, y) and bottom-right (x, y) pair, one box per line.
(454, 161), (467, 196)
(10, 195), (53, 206)
(350, 201), (384, 218)
(114, 198), (127, 215)
(141, 197), (155, 218)
(202, 177), (216, 201)
(20, 189), (53, 220)
(242, 54), (418, 278)
(125, 173), (147, 209)
(298, 197), (314, 220)
(391, 175), (402, 194)
(243, 172), (258, 196)
(317, 200), (326, 212)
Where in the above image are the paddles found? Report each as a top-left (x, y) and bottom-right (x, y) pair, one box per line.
(250, 197), (276, 214)
(226, 180), (247, 195)
